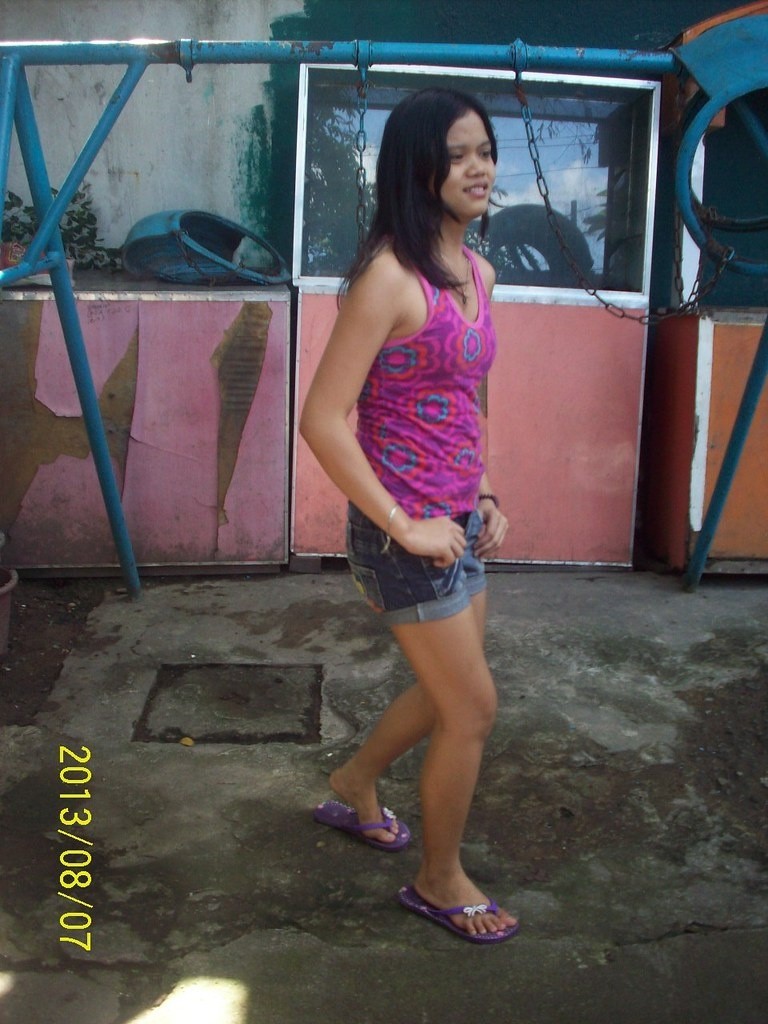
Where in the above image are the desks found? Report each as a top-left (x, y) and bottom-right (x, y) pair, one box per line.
(0, 270), (290, 579)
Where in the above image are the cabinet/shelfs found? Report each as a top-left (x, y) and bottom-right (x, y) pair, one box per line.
(287, 62), (663, 570)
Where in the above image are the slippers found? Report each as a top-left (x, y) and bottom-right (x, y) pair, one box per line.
(314, 800), (410, 851)
(399, 881), (519, 943)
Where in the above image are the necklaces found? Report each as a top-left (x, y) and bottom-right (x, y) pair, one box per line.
(445, 257), (470, 303)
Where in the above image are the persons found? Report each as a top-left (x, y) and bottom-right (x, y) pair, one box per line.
(298, 88), (520, 943)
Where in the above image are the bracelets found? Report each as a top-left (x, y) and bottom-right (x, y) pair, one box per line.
(379, 504), (400, 554)
(478, 492), (500, 508)
